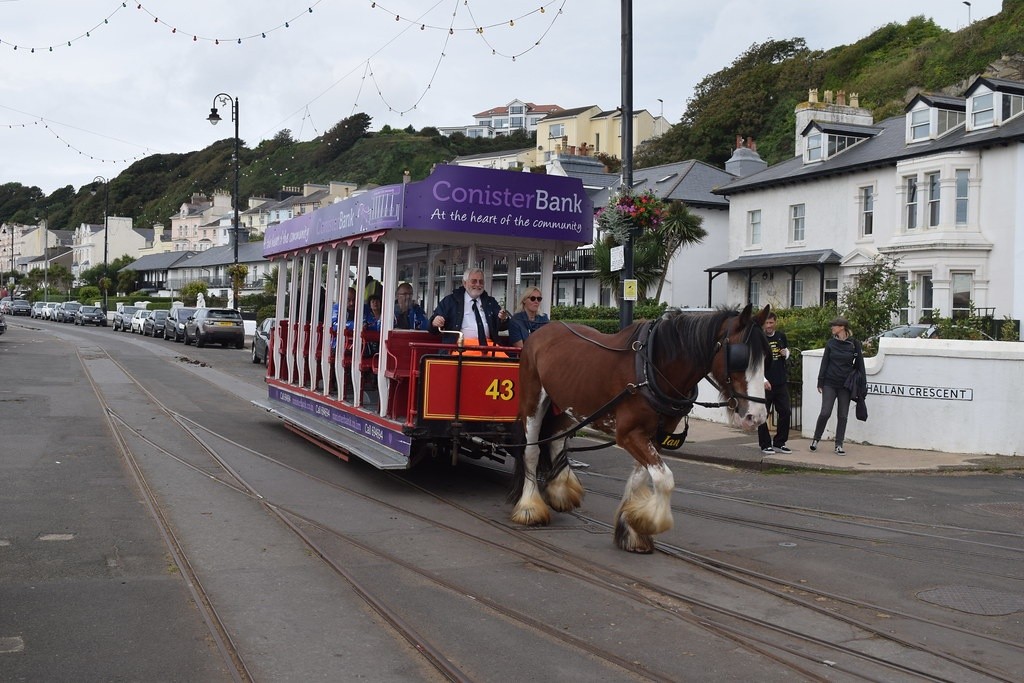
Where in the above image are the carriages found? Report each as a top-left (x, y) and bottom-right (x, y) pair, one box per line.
(251, 164), (773, 553)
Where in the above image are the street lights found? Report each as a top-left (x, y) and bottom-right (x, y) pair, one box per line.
(2, 220), (15, 301)
(33, 206), (47, 302)
(90, 176), (109, 317)
(206, 93), (239, 311)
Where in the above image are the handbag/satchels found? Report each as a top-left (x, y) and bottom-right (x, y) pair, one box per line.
(856, 396), (868, 422)
(844, 339), (862, 403)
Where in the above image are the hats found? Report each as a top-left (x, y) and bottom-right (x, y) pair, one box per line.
(829, 317), (848, 327)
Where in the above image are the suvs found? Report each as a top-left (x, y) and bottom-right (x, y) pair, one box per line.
(183, 307), (245, 348)
(9, 300), (31, 316)
(40, 302), (57, 320)
(30, 302), (47, 319)
(57, 302), (87, 323)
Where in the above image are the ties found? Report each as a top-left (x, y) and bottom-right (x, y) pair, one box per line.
(471, 298), (489, 355)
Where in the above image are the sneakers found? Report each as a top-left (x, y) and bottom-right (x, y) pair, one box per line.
(835, 445), (846, 456)
(773, 445), (792, 454)
(809, 439), (819, 452)
(761, 446), (775, 454)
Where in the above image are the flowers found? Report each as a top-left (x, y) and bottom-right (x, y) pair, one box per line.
(596, 183), (670, 231)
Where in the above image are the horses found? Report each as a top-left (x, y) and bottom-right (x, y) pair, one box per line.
(511, 302), (770, 554)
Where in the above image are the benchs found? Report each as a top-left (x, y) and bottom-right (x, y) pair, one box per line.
(279, 320), (510, 421)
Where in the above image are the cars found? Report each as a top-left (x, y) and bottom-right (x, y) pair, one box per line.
(163, 307), (197, 342)
(143, 309), (170, 338)
(0, 297), (11, 335)
(112, 306), (145, 332)
(74, 306), (107, 327)
(252, 318), (290, 368)
(660, 308), (721, 322)
(130, 310), (152, 334)
(862, 323), (997, 357)
(50, 303), (62, 321)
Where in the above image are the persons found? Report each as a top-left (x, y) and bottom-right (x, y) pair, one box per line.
(758, 312), (794, 454)
(292, 265), (428, 357)
(427, 267), (510, 358)
(808, 317), (866, 456)
(508, 286), (550, 353)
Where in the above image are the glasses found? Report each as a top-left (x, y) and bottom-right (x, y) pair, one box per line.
(467, 278), (485, 286)
(527, 295), (543, 303)
(399, 292), (414, 297)
